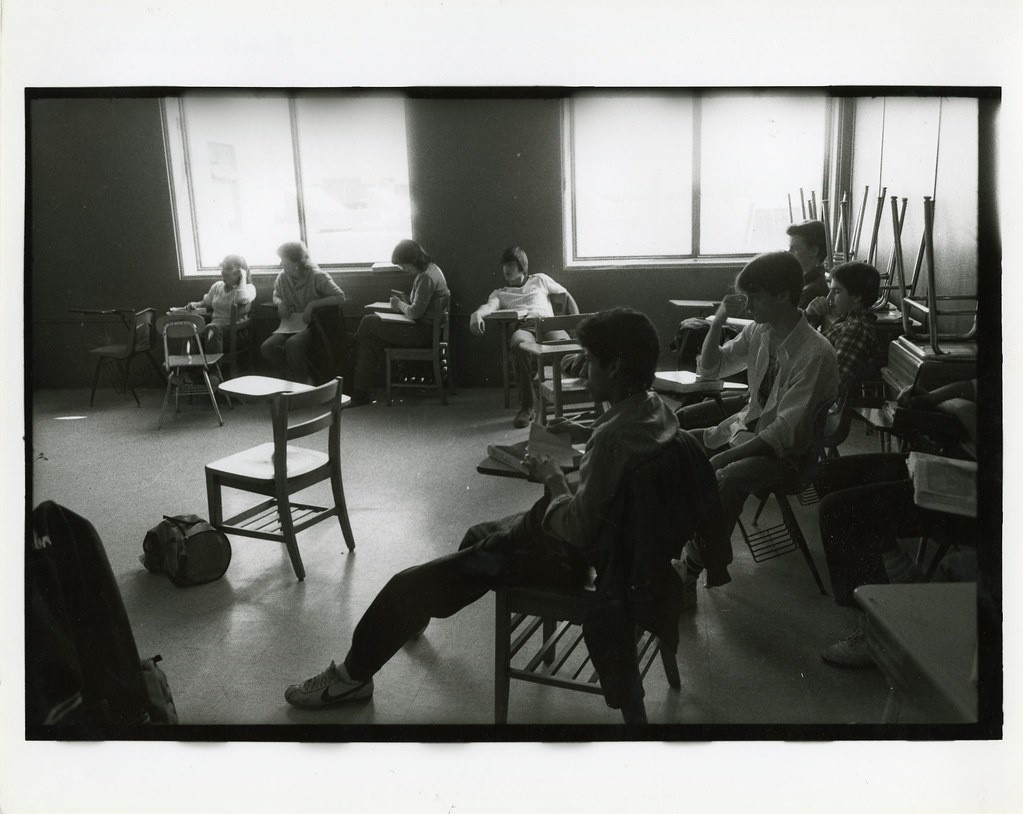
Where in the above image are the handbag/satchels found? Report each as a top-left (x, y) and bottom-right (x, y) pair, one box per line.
(141, 513), (231, 587)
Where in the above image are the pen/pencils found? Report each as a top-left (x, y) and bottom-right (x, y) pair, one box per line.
(289, 305), (295, 313)
(381, 286), (392, 296)
(553, 409), (591, 427)
(190, 303), (196, 312)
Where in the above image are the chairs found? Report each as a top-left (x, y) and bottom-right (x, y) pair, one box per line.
(70, 181), (977, 724)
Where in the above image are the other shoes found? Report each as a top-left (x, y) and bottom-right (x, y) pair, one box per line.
(514, 406), (536, 429)
(822, 623), (875, 669)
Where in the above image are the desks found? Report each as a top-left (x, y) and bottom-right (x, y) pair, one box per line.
(853, 580), (976, 725)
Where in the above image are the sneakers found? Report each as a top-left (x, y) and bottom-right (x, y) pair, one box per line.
(667, 558), (698, 610)
(285, 660), (373, 711)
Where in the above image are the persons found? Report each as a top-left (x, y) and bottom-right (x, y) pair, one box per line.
(346, 239), (448, 407)
(671, 262), (882, 432)
(468, 246), (585, 429)
(283, 307), (679, 708)
(673, 222), (830, 373)
(684, 252), (841, 579)
(183, 259), (259, 387)
(811, 373), (976, 672)
(257, 242), (347, 382)
